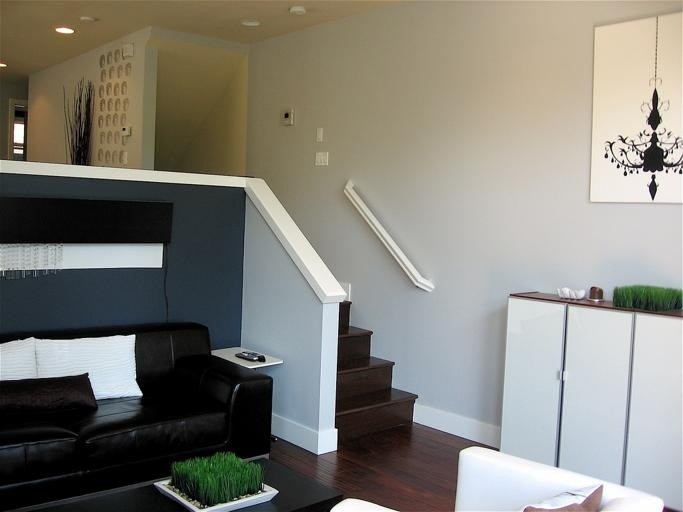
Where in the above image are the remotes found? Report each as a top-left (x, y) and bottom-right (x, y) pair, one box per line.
(235, 353), (259, 361)
(242, 351), (265, 362)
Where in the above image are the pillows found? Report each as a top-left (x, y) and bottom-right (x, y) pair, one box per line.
(0, 335), (39, 381)
(34, 332), (146, 403)
(0, 373), (99, 424)
(516, 485), (605, 512)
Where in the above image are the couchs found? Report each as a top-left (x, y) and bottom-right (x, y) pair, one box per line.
(0, 321), (274, 512)
(326, 445), (664, 512)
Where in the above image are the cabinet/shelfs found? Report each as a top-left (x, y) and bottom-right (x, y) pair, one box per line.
(621, 309), (681, 512)
(500, 292), (637, 491)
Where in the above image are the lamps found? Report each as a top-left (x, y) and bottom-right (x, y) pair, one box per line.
(602, 16), (682, 200)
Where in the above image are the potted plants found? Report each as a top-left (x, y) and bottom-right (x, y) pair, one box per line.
(151, 451), (279, 512)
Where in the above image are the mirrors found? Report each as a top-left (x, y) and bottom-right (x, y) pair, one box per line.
(588, 11), (682, 205)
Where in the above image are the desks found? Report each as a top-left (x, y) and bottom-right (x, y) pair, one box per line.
(210, 346), (282, 371)
